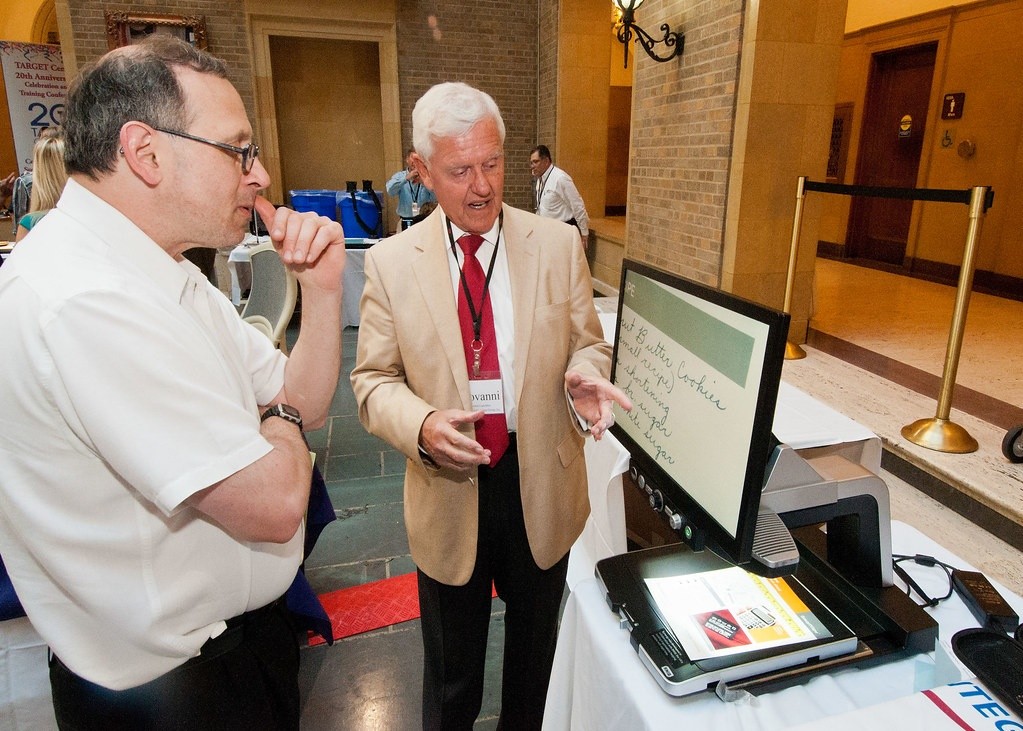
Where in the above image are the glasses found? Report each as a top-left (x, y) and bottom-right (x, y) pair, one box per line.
(120, 126), (258, 176)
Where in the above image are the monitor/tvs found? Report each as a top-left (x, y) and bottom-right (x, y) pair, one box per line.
(615, 259), (791, 565)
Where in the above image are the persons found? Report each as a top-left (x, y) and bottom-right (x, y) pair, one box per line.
(0, 31), (345, 731)
(530, 145), (591, 254)
(8, 127), (72, 251)
(384, 148), (438, 234)
(350, 83), (637, 731)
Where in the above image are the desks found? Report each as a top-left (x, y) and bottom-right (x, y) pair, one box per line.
(542, 519), (1022, 730)
(227, 235), (380, 331)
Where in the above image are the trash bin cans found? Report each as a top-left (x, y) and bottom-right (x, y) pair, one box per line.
(288, 189), (336, 221)
(336, 191), (384, 238)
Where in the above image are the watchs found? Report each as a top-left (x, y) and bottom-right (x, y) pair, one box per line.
(261, 403), (303, 430)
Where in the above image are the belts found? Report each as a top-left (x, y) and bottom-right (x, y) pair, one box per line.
(54, 594), (286, 680)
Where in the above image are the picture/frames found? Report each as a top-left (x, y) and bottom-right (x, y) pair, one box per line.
(103, 9), (208, 53)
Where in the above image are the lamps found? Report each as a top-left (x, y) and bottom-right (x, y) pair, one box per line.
(611, 0), (685, 69)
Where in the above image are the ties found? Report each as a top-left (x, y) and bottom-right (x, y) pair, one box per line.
(457, 234), (509, 469)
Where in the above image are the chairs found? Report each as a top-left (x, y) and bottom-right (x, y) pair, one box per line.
(239, 241), (297, 357)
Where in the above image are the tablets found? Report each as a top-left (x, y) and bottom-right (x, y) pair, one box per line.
(598, 541), (856, 698)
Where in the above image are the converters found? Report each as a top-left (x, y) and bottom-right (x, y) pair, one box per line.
(949, 569), (1019, 633)
